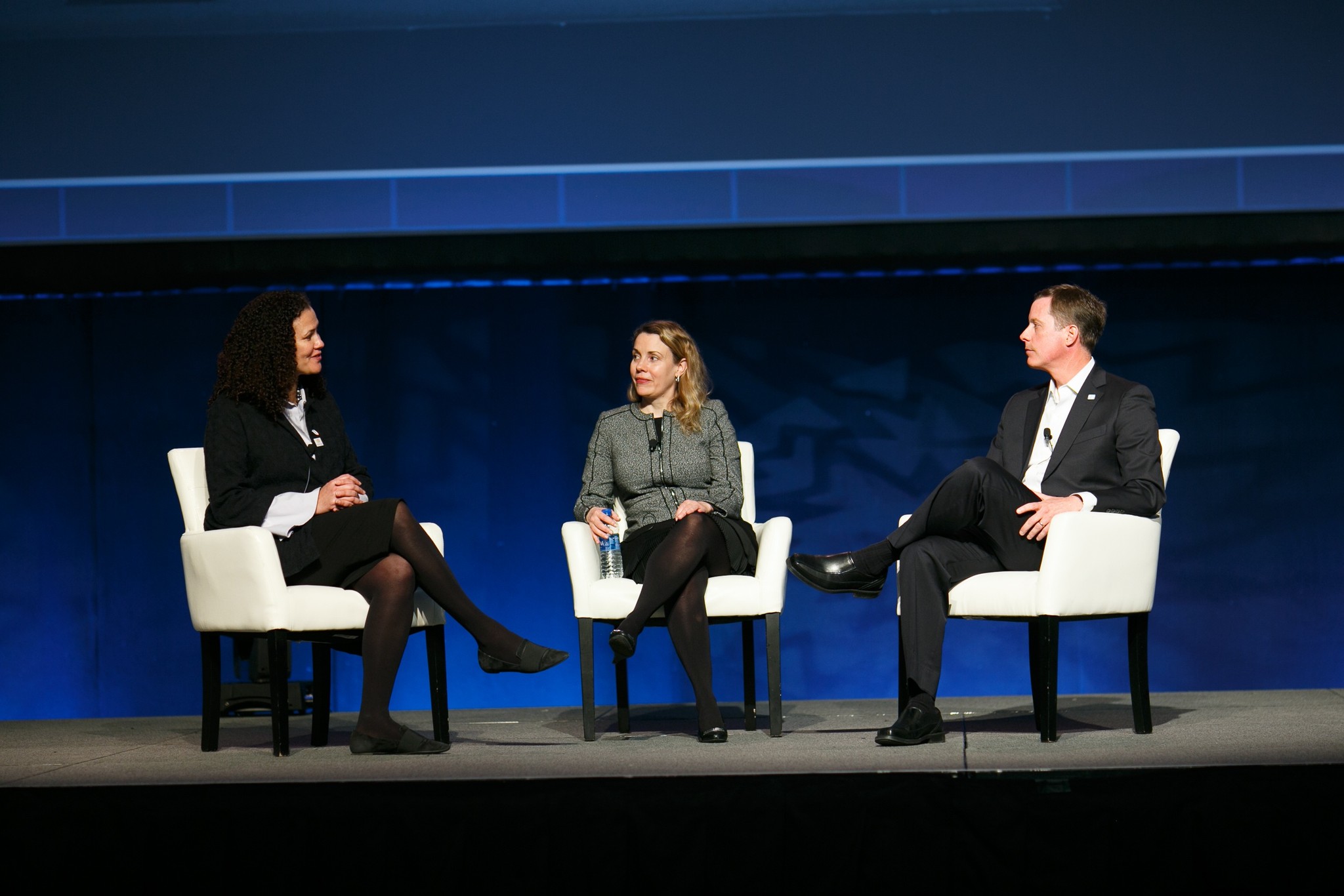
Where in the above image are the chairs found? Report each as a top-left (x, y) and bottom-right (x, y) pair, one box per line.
(896, 426), (1182, 742)
(167, 446), (449, 755)
(560, 440), (795, 743)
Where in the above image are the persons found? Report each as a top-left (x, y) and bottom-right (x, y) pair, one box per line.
(786, 284), (1166, 745)
(574, 319), (759, 743)
(203, 291), (570, 756)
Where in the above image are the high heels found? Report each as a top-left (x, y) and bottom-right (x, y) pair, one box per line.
(608, 629), (635, 663)
(696, 710), (728, 743)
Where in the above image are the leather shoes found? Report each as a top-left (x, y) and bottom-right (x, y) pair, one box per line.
(874, 702), (948, 744)
(786, 552), (886, 599)
(350, 725), (450, 755)
(478, 638), (569, 674)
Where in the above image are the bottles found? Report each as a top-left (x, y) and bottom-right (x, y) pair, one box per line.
(599, 509), (624, 579)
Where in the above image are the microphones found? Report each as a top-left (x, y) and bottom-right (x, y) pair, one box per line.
(308, 444), (314, 455)
(649, 439), (657, 452)
(1044, 428), (1051, 444)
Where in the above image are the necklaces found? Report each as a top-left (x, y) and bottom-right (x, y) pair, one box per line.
(296, 387), (301, 403)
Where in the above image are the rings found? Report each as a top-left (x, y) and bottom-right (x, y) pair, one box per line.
(1039, 521), (1045, 527)
